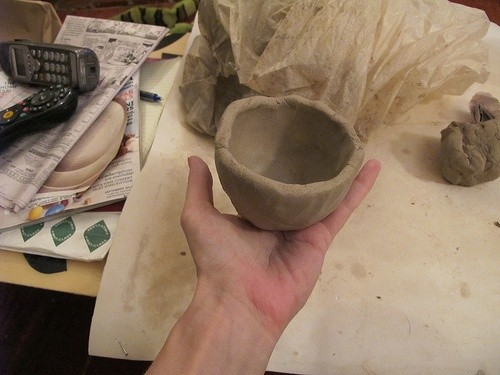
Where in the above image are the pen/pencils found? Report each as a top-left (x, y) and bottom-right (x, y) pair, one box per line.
(140, 89), (163, 101)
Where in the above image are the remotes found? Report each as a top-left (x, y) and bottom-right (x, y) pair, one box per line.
(0, 83), (78, 154)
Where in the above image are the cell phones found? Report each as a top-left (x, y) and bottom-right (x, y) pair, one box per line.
(0, 38), (100, 93)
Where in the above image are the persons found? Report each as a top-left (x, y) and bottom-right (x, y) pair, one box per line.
(141, 155), (381, 375)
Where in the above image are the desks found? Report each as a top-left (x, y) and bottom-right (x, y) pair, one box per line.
(1, 1), (499, 301)
